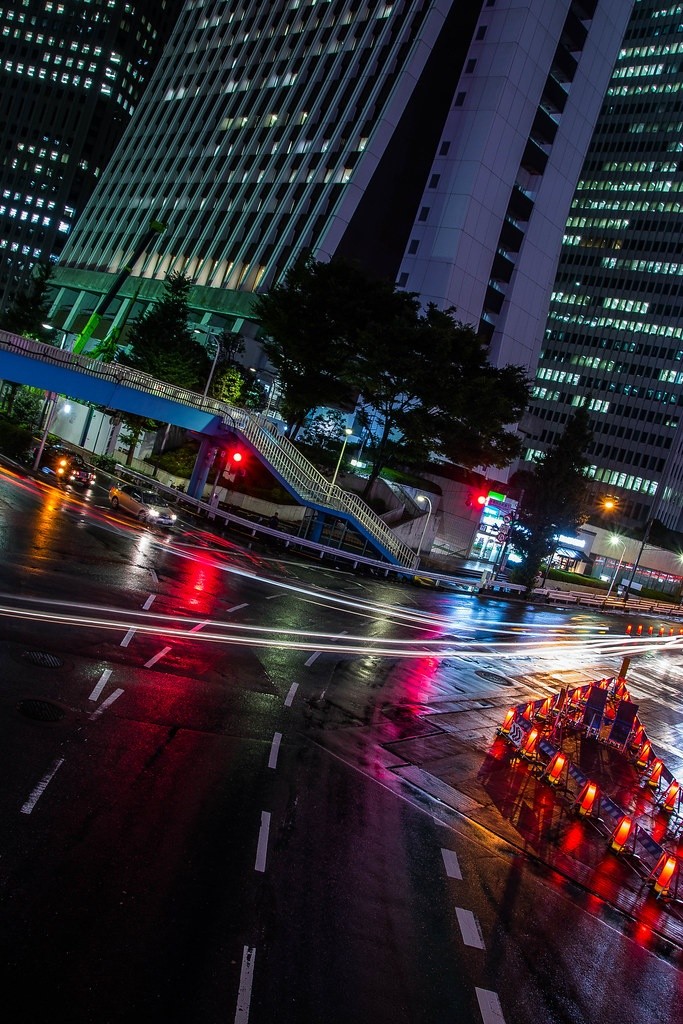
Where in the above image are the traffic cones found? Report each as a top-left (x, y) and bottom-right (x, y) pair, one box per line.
(625, 625), (683, 638)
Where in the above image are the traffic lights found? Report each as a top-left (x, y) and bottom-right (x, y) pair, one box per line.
(230, 450), (244, 474)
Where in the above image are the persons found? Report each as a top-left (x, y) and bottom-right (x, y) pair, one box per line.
(268, 512), (279, 529)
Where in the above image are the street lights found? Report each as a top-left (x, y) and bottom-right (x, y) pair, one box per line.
(601, 534), (626, 605)
(541, 524), (559, 589)
(195, 327), (219, 411)
(415, 495), (430, 556)
(325, 429), (353, 504)
(31, 319), (69, 473)
(478, 496), (513, 579)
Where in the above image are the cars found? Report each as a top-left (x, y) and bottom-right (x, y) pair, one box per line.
(109, 484), (178, 527)
(32, 444), (92, 489)
